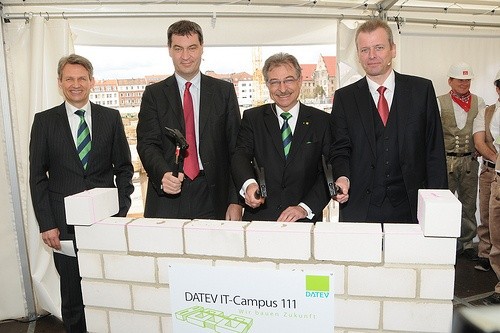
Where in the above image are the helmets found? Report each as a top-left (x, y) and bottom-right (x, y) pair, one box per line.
(448, 61), (473, 80)
(495, 70), (500, 84)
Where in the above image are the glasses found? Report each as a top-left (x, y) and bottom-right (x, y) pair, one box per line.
(268, 79), (299, 87)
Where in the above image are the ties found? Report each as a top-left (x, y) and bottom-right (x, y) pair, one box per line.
(376, 86), (390, 127)
(182, 82), (201, 180)
(279, 112), (293, 160)
(74, 109), (92, 170)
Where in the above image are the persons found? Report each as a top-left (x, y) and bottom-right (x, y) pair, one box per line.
(230, 52), (335, 222)
(28, 54), (134, 332)
(136, 19), (246, 222)
(473, 69), (500, 270)
(330, 18), (447, 225)
(436, 61), (490, 266)
(489, 149), (500, 294)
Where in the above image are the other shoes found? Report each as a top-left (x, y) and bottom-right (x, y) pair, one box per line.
(459, 245), (500, 294)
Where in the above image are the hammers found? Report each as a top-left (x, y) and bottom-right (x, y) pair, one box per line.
(253, 157), (267, 200)
(164, 126), (189, 179)
(321, 154), (343, 197)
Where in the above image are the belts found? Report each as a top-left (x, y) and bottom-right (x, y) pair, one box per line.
(182, 170), (206, 177)
(482, 160), (497, 169)
(445, 151), (472, 157)
